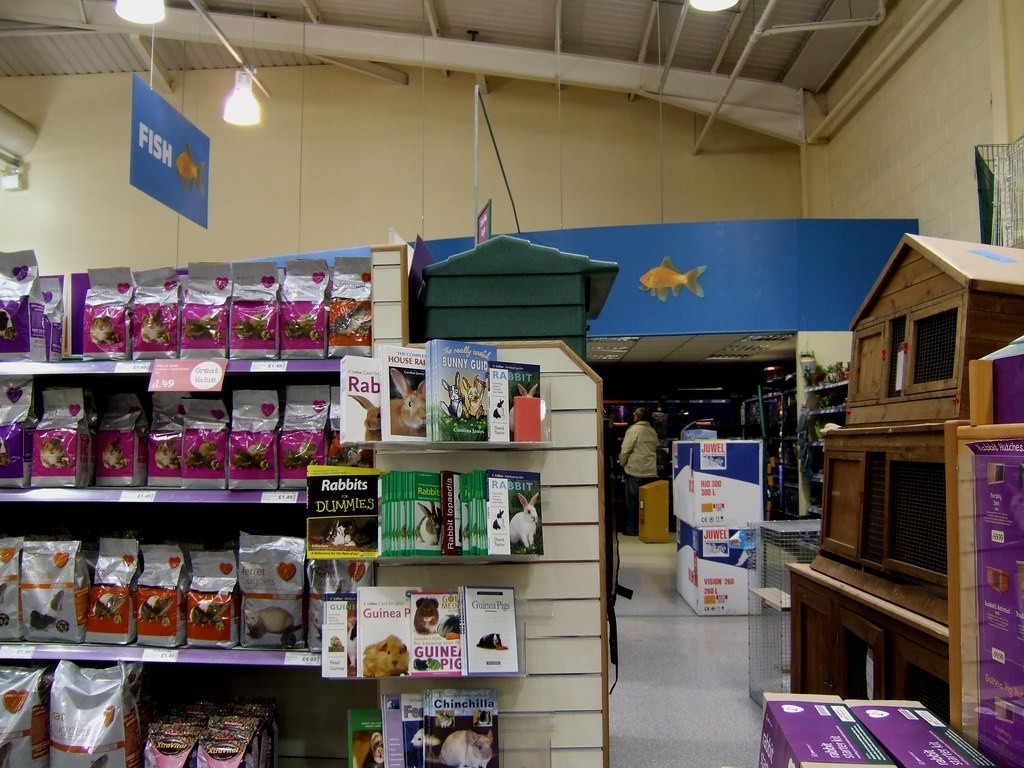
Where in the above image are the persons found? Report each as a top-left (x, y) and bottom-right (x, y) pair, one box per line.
(619, 408), (657, 537)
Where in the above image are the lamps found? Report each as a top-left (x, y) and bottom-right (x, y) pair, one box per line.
(114, 0), (271, 127)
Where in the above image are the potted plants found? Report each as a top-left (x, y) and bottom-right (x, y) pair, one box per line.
(812, 365), (828, 386)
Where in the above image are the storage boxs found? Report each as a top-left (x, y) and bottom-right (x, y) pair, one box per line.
(757, 328), (1023, 768)
(675, 518), (763, 616)
(672, 440), (765, 531)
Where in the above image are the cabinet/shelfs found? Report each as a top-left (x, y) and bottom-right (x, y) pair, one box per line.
(602, 377), (798, 533)
(805, 381), (849, 514)
(0, 235), (619, 767)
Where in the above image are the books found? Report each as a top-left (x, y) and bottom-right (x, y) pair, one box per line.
(306, 341), (543, 768)
